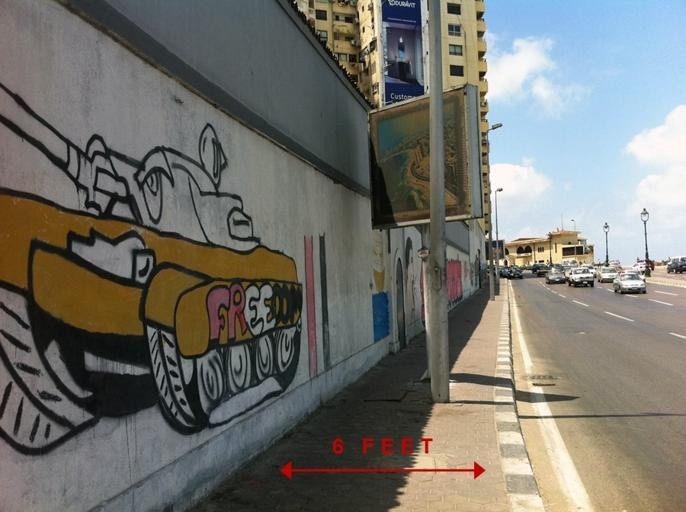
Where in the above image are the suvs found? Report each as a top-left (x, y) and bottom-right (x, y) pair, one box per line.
(667, 257), (686, 273)
(568, 268), (595, 287)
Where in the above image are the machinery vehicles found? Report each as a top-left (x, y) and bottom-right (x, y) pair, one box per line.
(0, 87), (302, 456)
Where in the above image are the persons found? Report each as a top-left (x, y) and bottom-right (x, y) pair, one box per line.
(598, 259), (601, 268)
(546, 259), (550, 267)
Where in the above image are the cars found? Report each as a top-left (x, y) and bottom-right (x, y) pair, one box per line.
(583, 261), (654, 294)
(487, 265), (523, 280)
(532, 263), (566, 283)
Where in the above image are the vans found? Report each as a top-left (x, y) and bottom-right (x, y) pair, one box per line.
(562, 259), (578, 272)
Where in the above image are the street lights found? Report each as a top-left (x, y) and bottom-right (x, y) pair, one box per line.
(604, 222), (609, 266)
(641, 208), (650, 275)
(571, 219), (577, 259)
(486, 124), (504, 300)
